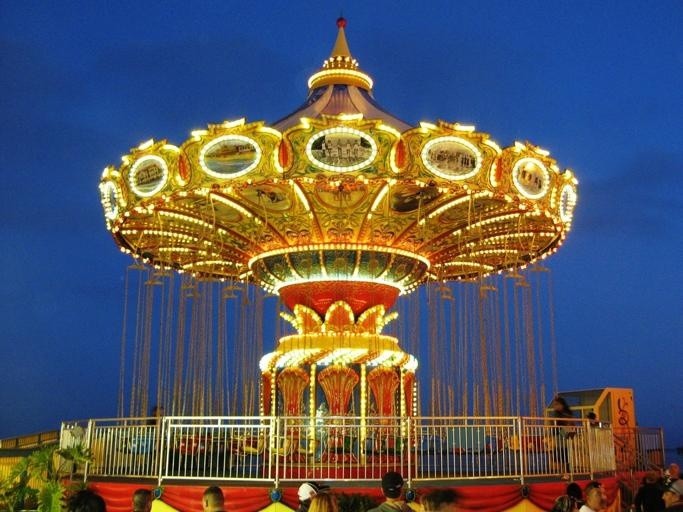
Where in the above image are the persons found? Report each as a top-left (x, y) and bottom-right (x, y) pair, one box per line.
(146, 405), (167, 425)
(552, 396), (576, 480)
(587, 412), (599, 426)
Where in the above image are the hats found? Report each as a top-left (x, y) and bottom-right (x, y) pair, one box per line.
(298, 482), (331, 502)
(382, 473), (404, 499)
